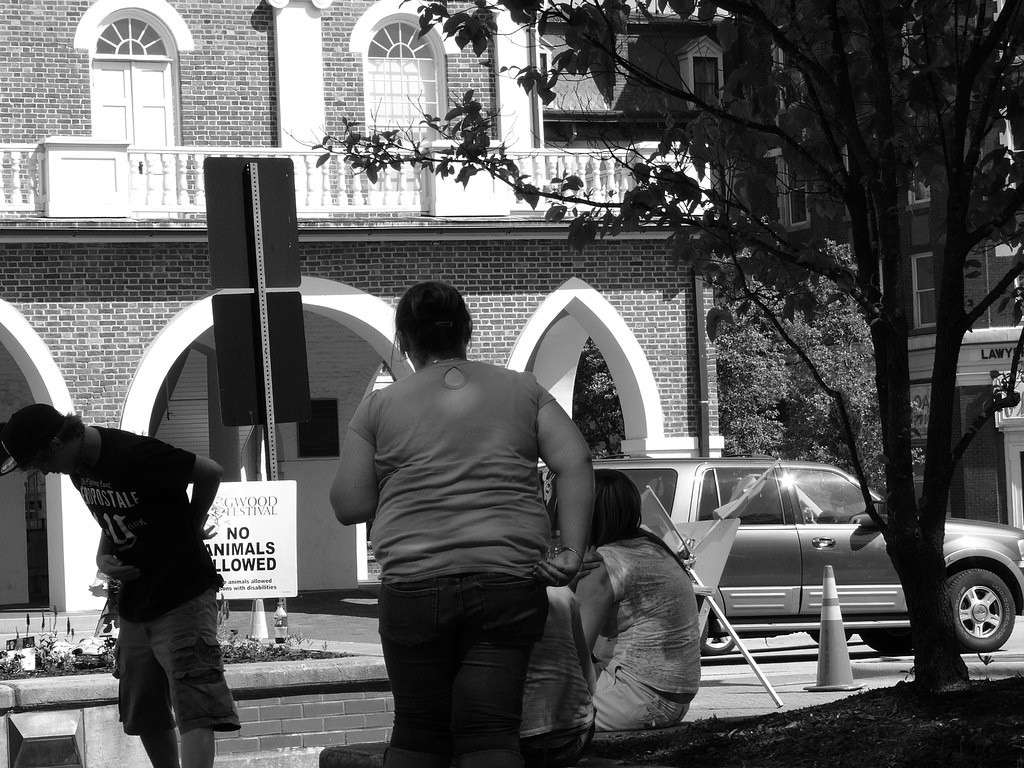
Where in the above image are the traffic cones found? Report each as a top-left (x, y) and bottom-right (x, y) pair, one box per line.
(249, 599), (269, 642)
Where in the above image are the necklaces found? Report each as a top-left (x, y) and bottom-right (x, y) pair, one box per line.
(433, 357), (466, 364)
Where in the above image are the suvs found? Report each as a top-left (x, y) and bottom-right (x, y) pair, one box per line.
(535, 450), (1024, 657)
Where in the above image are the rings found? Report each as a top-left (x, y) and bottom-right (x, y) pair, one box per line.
(555, 580), (560, 586)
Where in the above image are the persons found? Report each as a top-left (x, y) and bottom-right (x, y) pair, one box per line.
(570, 467), (700, 730)
(1, 403), (241, 768)
(518, 585), (596, 768)
(329, 283), (595, 768)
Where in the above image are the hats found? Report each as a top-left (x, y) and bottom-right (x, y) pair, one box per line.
(0, 404), (65, 475)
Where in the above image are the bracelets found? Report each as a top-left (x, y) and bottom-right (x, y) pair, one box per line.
(558, 547), (584, 572)
